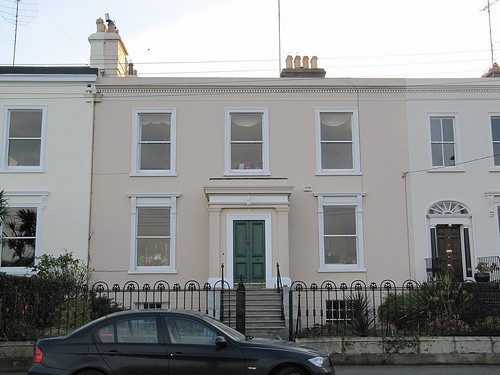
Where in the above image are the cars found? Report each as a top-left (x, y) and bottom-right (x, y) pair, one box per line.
(28, 308), (336, 375)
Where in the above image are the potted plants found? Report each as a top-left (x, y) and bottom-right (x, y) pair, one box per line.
(474, 262), (500, 282)
